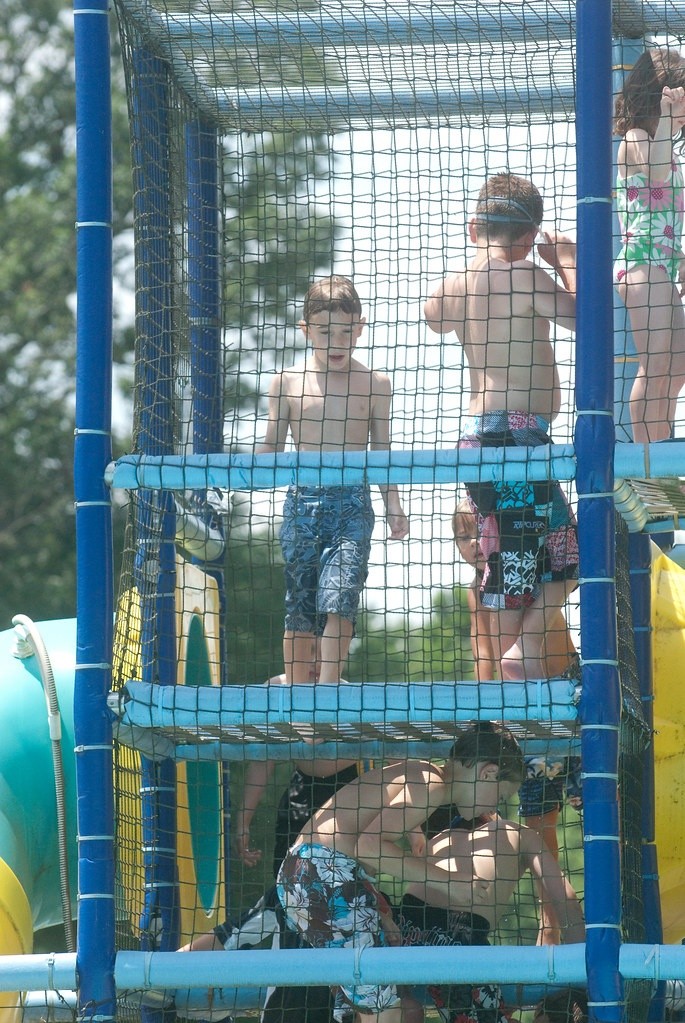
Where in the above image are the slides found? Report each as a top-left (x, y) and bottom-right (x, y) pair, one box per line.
(644, 551), (685, 950)
(0, 580), (227, 1022)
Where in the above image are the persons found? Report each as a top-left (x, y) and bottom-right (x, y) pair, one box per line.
(451, 499), (579, 678)
(116, 751), (582, 1023)
(425, 174), (577, 677)
(276, 722), (527, 1017)
(396, 813), (587, 1022)
(614, 48), (685, 444)
(255, 274), (410, 685)
